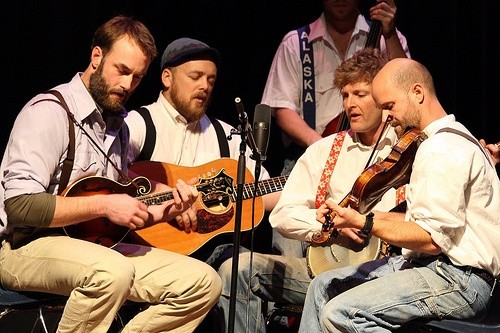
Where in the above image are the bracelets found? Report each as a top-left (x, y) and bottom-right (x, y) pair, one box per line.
(339, 228), (342, 232)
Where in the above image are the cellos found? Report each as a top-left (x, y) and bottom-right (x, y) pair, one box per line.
(317, 0), (390, 141)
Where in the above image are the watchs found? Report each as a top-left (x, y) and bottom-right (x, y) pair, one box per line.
(356, 212), (374, 237)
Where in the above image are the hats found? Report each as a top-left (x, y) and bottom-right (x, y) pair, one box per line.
(160, 38), (218, 70)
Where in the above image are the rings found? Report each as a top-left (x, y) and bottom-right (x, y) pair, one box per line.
(189, 204), (191, 206)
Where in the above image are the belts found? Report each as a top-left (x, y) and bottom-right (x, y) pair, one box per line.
(439, 254), (495, 288)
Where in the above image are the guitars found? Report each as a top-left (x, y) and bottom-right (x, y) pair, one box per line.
(61, 167), (233, 250)
(305, 143), (500, 283)
(126, 158), (295, 254)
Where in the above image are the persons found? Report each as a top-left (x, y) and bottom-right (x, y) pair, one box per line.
(0, 17), (221, 333)
(261, 0), (410, 317)
(215, 48), (500, 333)
(121, 38), (282, 333)
(298, 58), (484, 333)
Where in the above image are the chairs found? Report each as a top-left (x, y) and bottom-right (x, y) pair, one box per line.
(418, 286), (500, 333)
(0, 286), (68, 333)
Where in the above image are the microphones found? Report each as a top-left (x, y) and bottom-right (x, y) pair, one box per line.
(252, 104), (271, 179)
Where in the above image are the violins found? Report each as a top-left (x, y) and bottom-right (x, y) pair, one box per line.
(309, 129), (425, 243)
